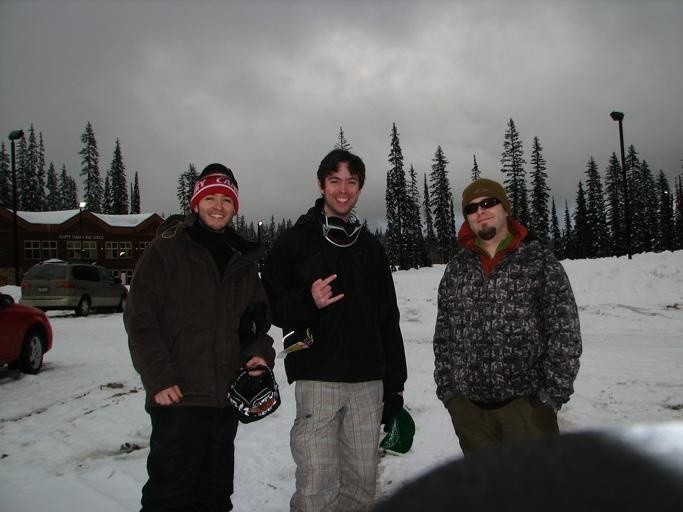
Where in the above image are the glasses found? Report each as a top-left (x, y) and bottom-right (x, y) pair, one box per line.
(226, 365), (281, 424)
(464, 198), (502, 214)
(323, 213), (363, 248)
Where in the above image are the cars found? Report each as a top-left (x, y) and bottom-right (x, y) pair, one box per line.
(0, 291), (52, 375)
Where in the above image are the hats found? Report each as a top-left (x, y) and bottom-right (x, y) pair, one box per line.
(462, 179), (511, 220)
(190, 164), (239, 214)
(379, 408), (415, 454)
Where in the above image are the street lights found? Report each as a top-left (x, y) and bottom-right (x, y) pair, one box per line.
(117, 251), (126, 278)
(664, 191), (674, 253)
(609, 111), (632, 260)
(257, 221), (263, 272)
(78, 202), (86, 263)
(7, 129), (24, 286)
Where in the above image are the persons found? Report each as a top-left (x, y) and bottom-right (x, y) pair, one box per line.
(262, 149), (407, 511)
(433, 178), (581, 510)
(124, 163), (276, 511)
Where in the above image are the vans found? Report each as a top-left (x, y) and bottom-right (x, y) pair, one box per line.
(17, 262), (128, 316)
